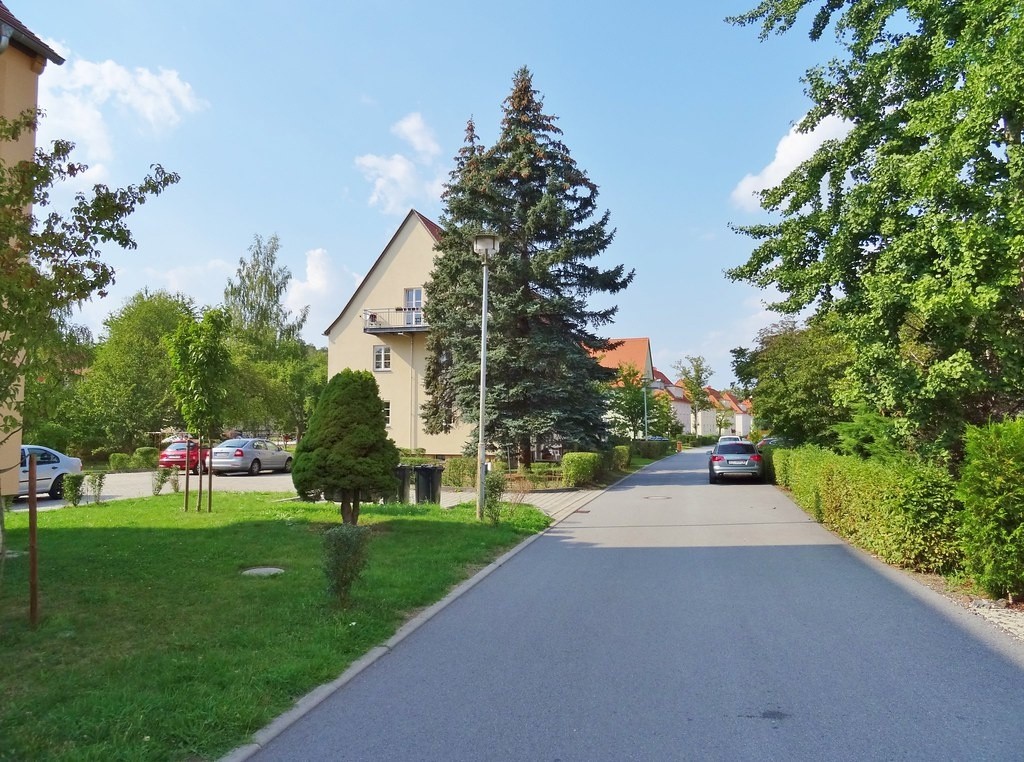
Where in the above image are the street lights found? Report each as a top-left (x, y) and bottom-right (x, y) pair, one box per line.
(644, 379), (662, 441)
(472, 231), (503, 519)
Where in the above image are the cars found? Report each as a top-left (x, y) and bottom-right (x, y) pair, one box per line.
(756, 438), (793, 449)
(208, 437), (294, 476)
(718, 436), (742, 442)
(706, 439), (763, 484)
(19, 444), (83, 500)
(159, 439), (210, 475)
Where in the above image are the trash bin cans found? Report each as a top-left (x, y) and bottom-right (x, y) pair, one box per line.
(394, 464), (414, 504)
(414, 464), (446, 505)
